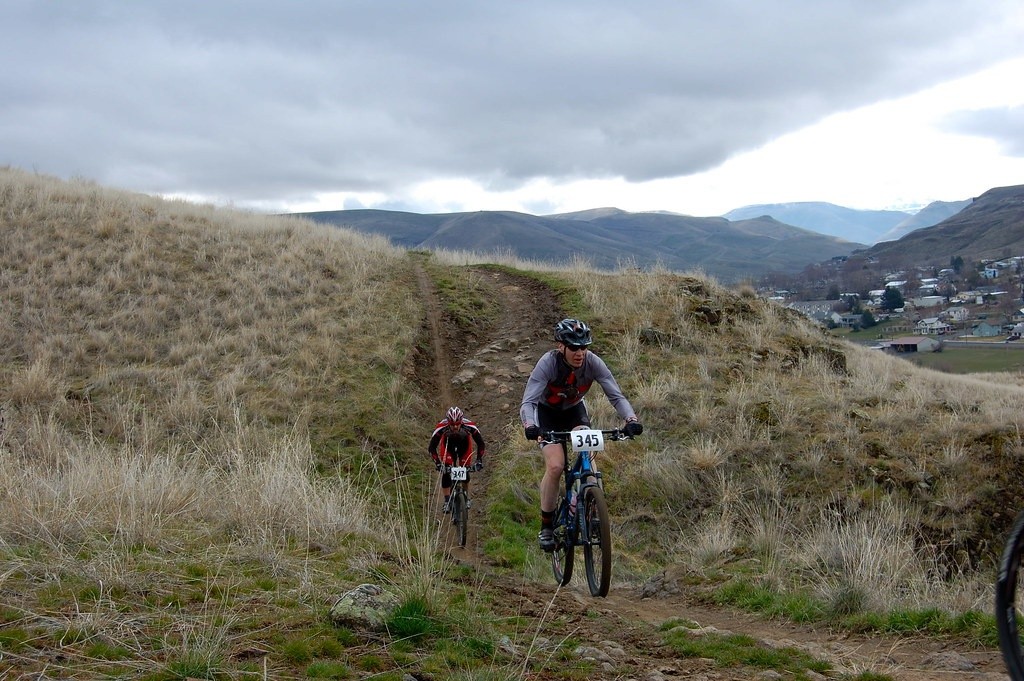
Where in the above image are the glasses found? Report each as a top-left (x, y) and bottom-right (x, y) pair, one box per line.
(563, 344), (588, 352)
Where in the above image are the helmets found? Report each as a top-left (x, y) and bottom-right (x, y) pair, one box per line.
(554, 318), (593, 345)
(445, 406), (464, 425)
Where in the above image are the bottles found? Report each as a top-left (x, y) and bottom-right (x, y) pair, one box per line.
(568, 488), (578, 523)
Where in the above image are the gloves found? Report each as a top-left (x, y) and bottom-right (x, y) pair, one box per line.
(473, 460), (482, 472)
(624, 420), (643, 440)
(433, 459), (443, 471)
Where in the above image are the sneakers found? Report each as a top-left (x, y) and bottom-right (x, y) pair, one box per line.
(537, 528), (553, 550)
(465, 499), (471, 509)
(442, 502), (450, 514)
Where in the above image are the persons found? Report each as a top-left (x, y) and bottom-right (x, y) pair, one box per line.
(428, 407), (486, 513)
(520, 319), (638, 551)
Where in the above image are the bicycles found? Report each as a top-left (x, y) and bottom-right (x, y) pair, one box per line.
(533, 428), (636, 598)
(438, 466), (484, 549)
